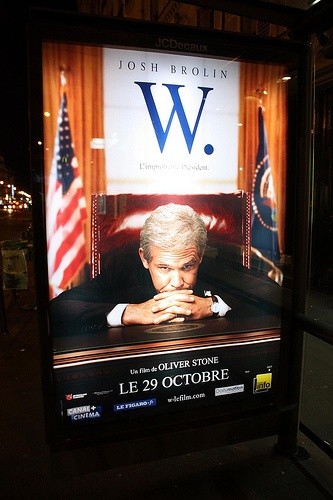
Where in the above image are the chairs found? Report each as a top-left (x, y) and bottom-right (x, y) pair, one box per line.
(89, 191), (252, 279)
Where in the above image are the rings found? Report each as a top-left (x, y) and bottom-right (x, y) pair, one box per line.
(176, 313), (182, 317)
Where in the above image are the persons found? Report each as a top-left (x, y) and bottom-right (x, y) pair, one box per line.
(48, 202), (281, 337)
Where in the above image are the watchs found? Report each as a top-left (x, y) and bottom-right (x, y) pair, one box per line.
(210, 296), (221, 319)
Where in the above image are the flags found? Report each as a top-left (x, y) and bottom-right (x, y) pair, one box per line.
(45, 89), (89, 296)
(249, 105), (282, 257)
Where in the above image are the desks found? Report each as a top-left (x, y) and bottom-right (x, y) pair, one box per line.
(46, 313), (282, 373)
